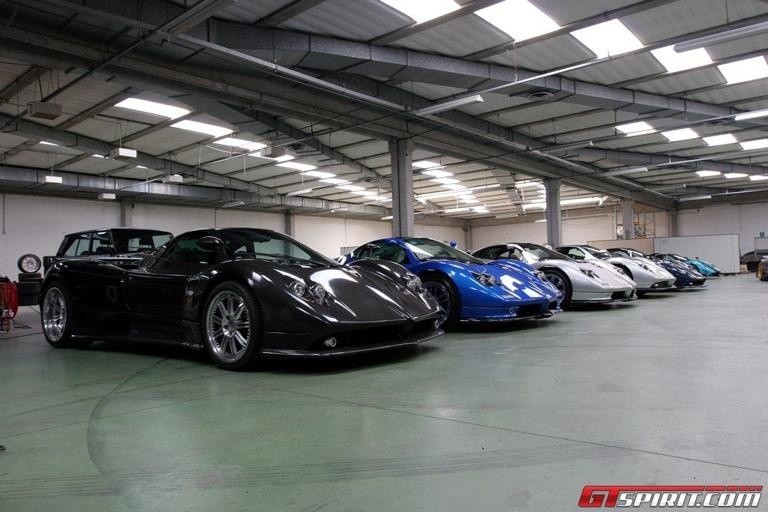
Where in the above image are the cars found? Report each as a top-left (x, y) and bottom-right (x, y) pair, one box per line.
(41, 227), (174, 277)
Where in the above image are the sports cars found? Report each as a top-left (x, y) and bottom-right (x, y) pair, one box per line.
(470, 242), (721, 310)
(37, 228), (449, 372)
(333, 235), (564, 333)
